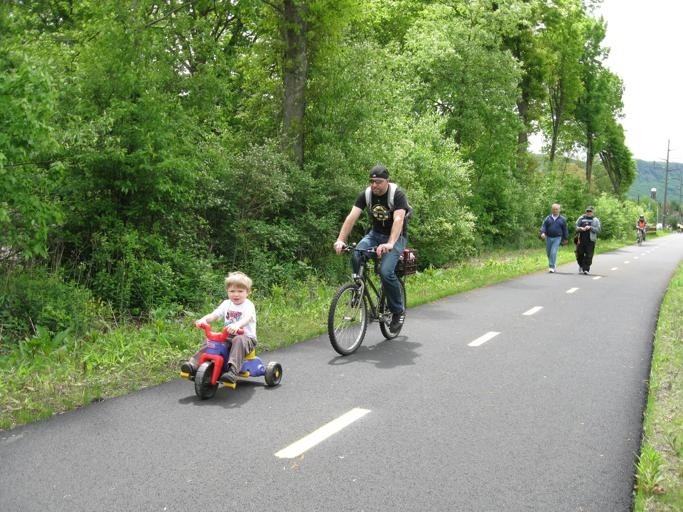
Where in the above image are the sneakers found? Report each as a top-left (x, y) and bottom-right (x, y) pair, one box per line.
(346, 299), (364, 308)
(579, 266), (589, 275)
(390, 313), (406, 329)
(180, 362), (194, 375)
(220, 371), (236, 383)
(549, 264), (556, 273)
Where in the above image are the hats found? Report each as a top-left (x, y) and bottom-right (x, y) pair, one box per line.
(586, 206), (594, 212)
(367, 166), (390, 181)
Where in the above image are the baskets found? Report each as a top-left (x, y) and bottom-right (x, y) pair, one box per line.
(373, 249), (417, 276)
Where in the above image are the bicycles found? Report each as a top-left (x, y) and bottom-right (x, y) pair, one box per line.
(325, 242), (415, 357)
(635, 226), (646, 246)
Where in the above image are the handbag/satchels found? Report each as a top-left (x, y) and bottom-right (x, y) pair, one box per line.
(574, 235), (578, 245)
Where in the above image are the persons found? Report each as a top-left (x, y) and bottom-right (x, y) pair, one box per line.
(179, 269), (258, 385)
(573, 205), (601, 276)
(331, 166), (411, 332)
(538, 203), (569, 273)
(634, 214), (648, 243)
